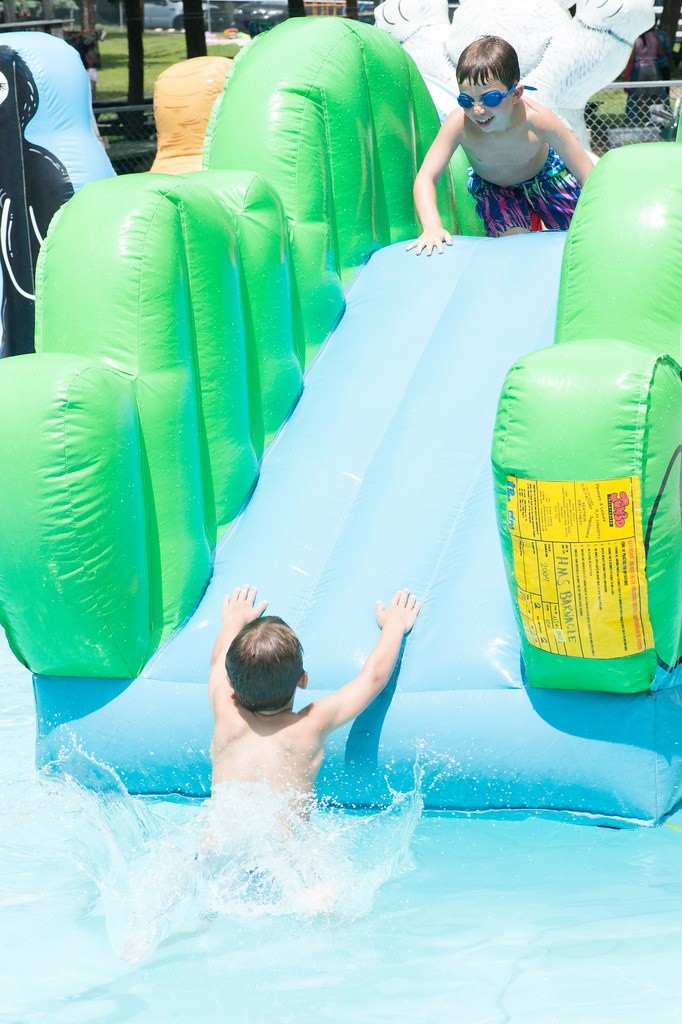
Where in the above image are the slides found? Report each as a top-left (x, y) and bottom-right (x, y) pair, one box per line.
(0, 12), (682, 829)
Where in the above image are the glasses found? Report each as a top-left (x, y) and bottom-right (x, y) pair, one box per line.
(457, 86), (537, 108)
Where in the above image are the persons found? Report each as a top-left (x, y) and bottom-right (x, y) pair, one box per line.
(406, 34), (595, 257)
(195, 582), (423, 907)
(628, 30), (664, 127)
(654, 27), (673, 95)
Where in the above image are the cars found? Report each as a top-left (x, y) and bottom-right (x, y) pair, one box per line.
(233, 1), (343, 39)
(144, 1), (219, 32)
